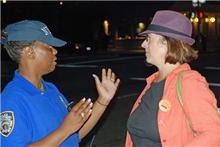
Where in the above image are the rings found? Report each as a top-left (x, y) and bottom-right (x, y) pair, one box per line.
(80, 112), (83, 118)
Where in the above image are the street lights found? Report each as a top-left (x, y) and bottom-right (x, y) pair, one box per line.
(191, 0), (206, 42)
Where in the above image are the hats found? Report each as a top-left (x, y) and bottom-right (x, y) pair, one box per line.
(1, 19), (67, 49)
(137, 10), (195, 46)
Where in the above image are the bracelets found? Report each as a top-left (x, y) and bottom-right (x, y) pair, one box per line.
(96, 100), (108, 107)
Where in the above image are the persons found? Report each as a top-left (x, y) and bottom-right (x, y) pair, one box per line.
(122, 9), (220, 147)
(1, 20), (120, 147)
